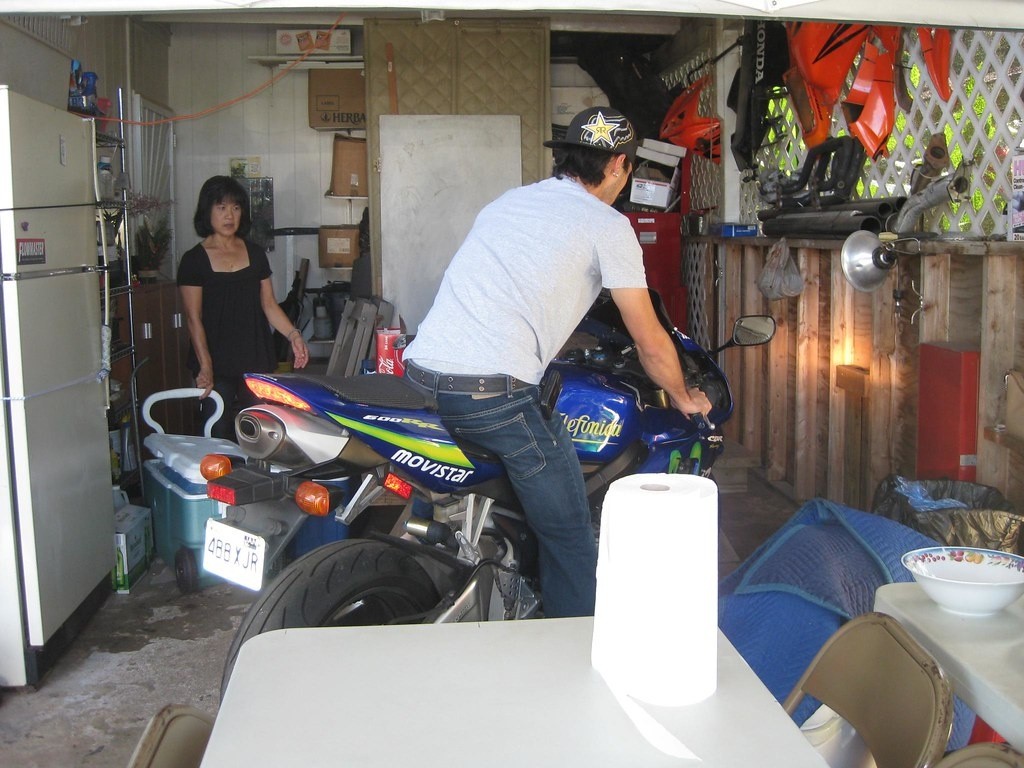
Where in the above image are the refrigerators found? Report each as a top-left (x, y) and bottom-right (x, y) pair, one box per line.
(1, 83), (122, 690)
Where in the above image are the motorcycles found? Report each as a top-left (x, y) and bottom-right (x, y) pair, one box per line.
(199, 288), (777, 699)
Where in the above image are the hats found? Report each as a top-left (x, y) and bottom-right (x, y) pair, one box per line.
(542, 106), (638, 163)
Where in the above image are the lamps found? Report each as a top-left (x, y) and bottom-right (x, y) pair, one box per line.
(841, 229), (933, 324)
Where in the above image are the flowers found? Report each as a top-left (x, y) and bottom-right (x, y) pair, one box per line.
(135, 215), (175, 270)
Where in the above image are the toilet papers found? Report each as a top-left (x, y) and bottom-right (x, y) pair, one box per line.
(591, 472), (718, 762)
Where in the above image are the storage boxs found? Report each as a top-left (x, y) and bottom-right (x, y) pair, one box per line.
(276, 29), (352, 54)
(713, 222), (758, 237)
(628, 137), (687, 210)
(307, 68), (366, 132)
(138, 433), (287, 593)
(319, 224), (360, 267)
(330, 133), (367, 196)
(109, 505), (154, 595)
(374, 315), (409, 376)
(1010, 146), (1024, 243)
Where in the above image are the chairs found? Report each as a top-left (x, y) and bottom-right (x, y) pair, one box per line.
(780, 614), (953, 768)
(931, 743), (1024, 768)
(129, 702), (215, 768)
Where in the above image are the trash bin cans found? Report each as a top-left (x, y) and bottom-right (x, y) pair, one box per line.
(870, 473), (1024, 559)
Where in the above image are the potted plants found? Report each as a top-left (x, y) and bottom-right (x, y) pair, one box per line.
(138, 268), (158, 284)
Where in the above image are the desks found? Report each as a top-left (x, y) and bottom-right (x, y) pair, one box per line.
(875, 583), (1024, 754)
(200, 616), (831, 767)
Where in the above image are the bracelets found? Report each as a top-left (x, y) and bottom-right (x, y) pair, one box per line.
(286, 329), (301, 340)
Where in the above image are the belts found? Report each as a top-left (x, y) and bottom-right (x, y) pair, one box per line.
(406, 359), (531, 394)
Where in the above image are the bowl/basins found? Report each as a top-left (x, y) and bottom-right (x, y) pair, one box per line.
(901, 547), (1024, 617)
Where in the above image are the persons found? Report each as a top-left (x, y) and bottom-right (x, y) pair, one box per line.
(400, 106), (712, 618)
(176, 175), (308, 444)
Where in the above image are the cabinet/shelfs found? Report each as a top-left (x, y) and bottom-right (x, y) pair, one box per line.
(114, 281), (202, 459)
(95, 130), (148, 508)
